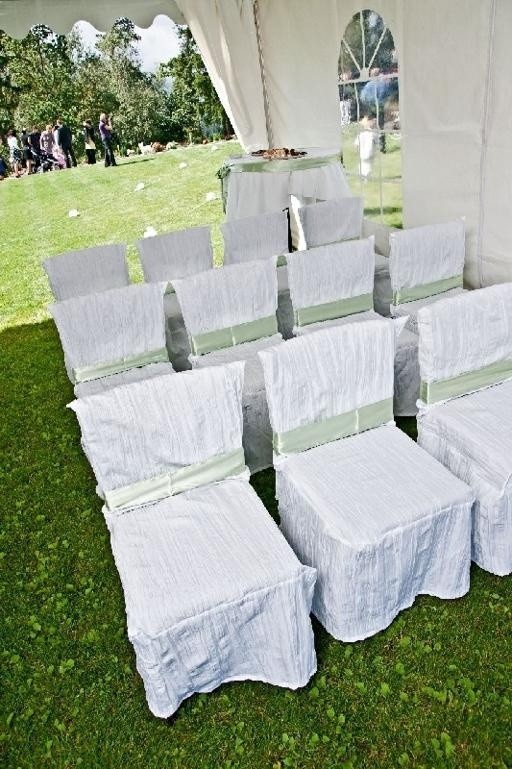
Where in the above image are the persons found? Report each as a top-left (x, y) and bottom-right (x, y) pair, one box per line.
(40, 122), (56, 173)
(359, 67), (395, 155)
(19, 126), (32, 175)
(83, 118), (97, 164)
(29, 125), (43, 173)
(338, 72), (352, 126)
(51, 125), (68, 169)
(98, 111), (122, 168)
(54, 119), (77, 169)
(7, 127), (23, 178)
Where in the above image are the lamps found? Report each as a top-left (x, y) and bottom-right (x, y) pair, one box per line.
(260, 316), (478, 643)
(42, 241), (130, 303)
(137, 224), (216, 372)
(61, 359), (323, 724)
(281, 235), (422, 420)
(220, 214), (296, 338)
(297, 193), (391, 311)
(170, 256), (285, 477)
(387, 217), (479, 321)
(413, 279), (512, 582)
(47, 279), (178, 402)
